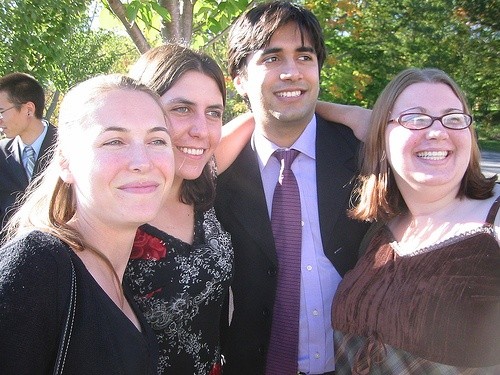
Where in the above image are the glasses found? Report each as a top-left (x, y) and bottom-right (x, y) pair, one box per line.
(0, 105), (16, 119)
(388, 112), (473, 130)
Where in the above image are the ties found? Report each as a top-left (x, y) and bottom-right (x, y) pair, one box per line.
(265, 149), (302, 375)
(24, 147), (35, 184)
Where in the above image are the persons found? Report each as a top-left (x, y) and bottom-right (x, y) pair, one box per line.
(0, 74), (176, 375)
(215, 2), (372, 375)
(331, 69), (500, 375)
(0, 73), (57, 247)
(124, 44), (373, 375)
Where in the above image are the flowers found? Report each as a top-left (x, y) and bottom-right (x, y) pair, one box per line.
(129, 229), (166, 262)
